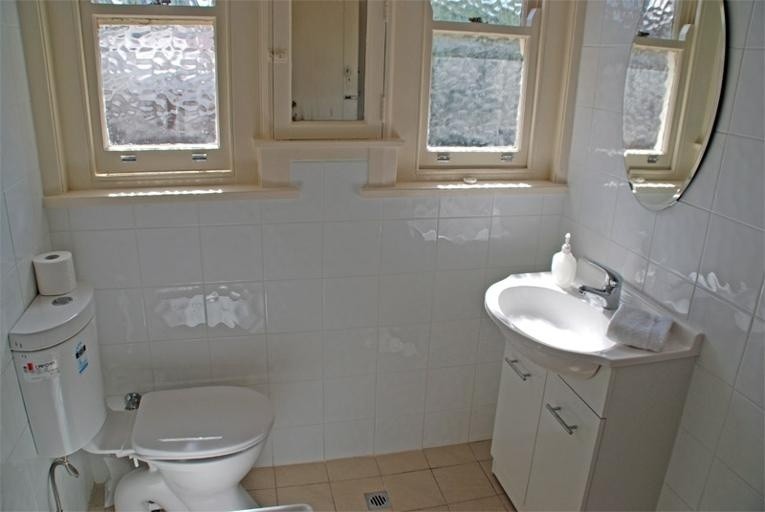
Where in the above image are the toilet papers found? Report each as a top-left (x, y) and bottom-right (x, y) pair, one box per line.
(34, 249), (77, 296)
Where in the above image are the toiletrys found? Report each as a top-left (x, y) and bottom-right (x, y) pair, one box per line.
(551, 233), (576, 288)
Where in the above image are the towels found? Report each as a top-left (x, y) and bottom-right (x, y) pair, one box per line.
(603, 301), (678, 353)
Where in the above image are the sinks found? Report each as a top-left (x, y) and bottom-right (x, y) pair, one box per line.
(484, 270), (618, 363)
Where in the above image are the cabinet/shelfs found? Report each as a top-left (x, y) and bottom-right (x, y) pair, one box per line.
(487, 336), (705, 511)
(256, 1), (395, 141)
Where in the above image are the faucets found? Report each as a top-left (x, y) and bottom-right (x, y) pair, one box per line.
(578, 256), (622, 309)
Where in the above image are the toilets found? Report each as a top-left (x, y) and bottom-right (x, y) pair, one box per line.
(8, 283), (273, 512)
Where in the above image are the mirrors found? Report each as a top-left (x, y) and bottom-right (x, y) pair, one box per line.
(620, 0), (732, 221)
(291, 1), (363, 122)
